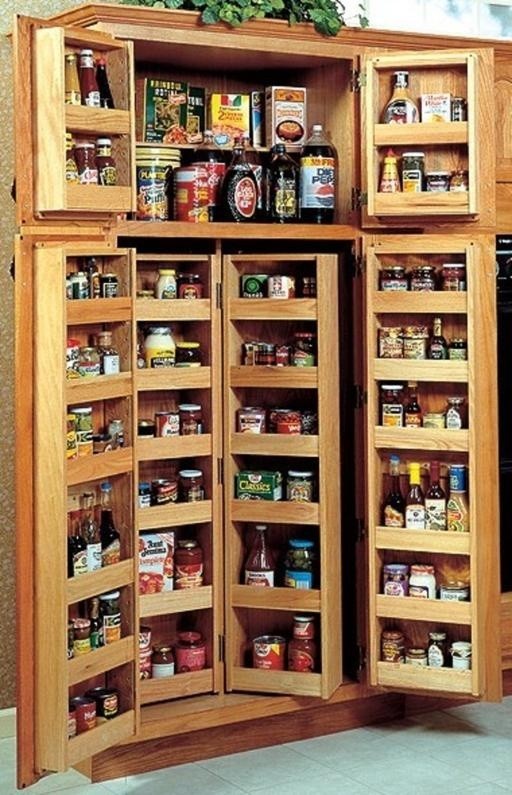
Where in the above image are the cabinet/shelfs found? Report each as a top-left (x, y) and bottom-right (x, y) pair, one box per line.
(1, 0), (512, 794)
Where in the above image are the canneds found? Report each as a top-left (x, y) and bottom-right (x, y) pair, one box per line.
(173, 538), (203, 589)
(286, 470), (312, 502)
(137, 403), (208, 436)
(401, 151), (468, 192)
(293, 333), (316, 365)
(285, 539), (313, 590)
(69, 685), (119, 738)
(237, 407), (303, 433)
(76, 138), (117, 185)
(423, 395), (464, 430)
(241, 274), (298, 299)
(252, 616), (315, 673)
(450, 98), (465, 120)
(448, 337), (466, 358)
(450, 464), (465, 491)
(135, 268), (202, 300)
(81, 348), (100, 375)
(384, 563), (468, 602)
(64, 406), (126, 457)
(379, 386), (405, 426)
(380, 264), (468, 291)
(64, 272), (89, 300)
(100, 272), (117, 298)
(140, 628), (207, 681)
(382, 629), (470, 669)
(139, 469), (205, 506)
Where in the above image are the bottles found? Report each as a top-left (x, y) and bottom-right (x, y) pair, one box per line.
(430, 314), (448, 359)
(69, 482), (121, 577)
(86, 257), (102, 299)
(452, 95), (466, 123)
(378, 149), (468, 195)
(108, 417), (125, 452)
(404, 379), (423, 428)
(65, 413), (79, 462)
(88, 597), (104, 652)
(239, 525), (276, 589)
(220, 123), (339, 226)
(378, 447), (474, 532)
(381, 70), (420, 126)
(282, 539), (316, 588)
(64, 48), (117, 186)
